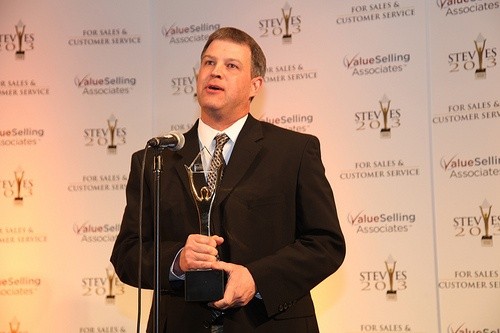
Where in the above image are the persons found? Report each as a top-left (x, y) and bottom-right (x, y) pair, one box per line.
(110, 27), (346, 333)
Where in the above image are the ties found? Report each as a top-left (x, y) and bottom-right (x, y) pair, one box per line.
(205, 133), (230, 197)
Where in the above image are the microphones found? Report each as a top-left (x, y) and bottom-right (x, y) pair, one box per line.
(147, 131), (185, 151)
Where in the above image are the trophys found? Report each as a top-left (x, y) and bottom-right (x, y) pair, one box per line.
(184, 145), (224, 302)
